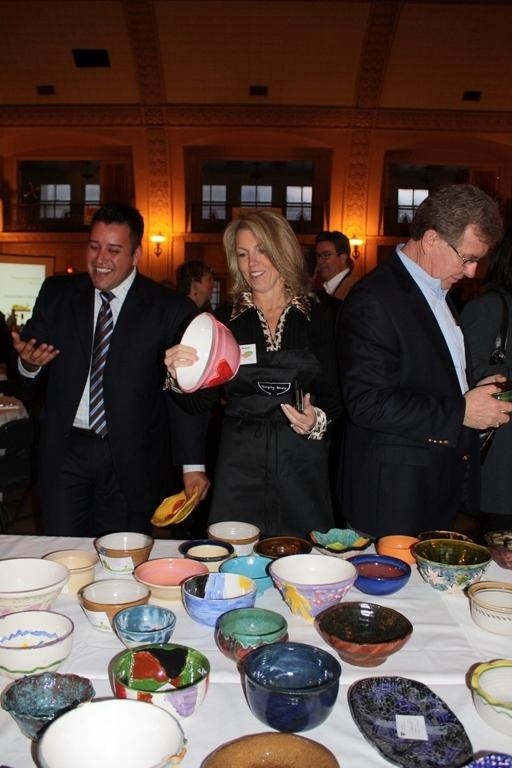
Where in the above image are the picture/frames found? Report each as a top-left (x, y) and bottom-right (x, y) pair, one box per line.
(0, 252), (55, 333)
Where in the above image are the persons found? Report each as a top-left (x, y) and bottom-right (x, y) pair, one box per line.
(173, 262), (215, 312)
(315, 233), (361, 299)
(163, 210), (346, 540)
(338, 184), (510, 535)
(11, 200), (212, 536)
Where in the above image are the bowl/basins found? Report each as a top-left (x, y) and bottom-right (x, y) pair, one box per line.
(177, 539), (234, 572)
(79, 579), (153, 633)
(470, 660), (512, 738)
(270, 554), (357, 617)
(200, 732), (341, 768)
(254, 537), (314, 562)
(36, 698), (188, 768)
(93, 532), (155, 575)
(0, 557), (70, 614)
(377, 536), (420, 567)
(344, 554), (411, 597)
(134, 556), (210, 601)
(462, 750), (511, 768)
(490, 385), (512, 413)
(410, 529), (511, 590)
(114, 605), (177, 650)
(219, 556), (274, 598)
(214, 607), (287, 664)
(182, 572), (257, 627)
(468, 579), (512, 637)
(173, 310), (243, 393)
(43, 549), (100, 594)
(112, 644), (212, 720)
(309, 528), (375, 560)
(313, 602), (413, 667)
(239, 642), (343, 734)
(1, 672), (97, 742)
(206, 522), (261, 557)
(2, 611), (74, 678)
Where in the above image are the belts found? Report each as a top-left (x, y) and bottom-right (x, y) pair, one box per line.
(68, 426), (110, 441)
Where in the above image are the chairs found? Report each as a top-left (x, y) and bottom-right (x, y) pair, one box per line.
(1, 415), (43, 536)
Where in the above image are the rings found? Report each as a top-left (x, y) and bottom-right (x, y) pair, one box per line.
(494, 421), (501, 429)
(291, 423), (295, 428)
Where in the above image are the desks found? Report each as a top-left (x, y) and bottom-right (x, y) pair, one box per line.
(1, 394), (28, 451)
(0, 525), (509, 768)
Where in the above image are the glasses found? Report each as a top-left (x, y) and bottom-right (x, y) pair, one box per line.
(312, 251), (339, 261)
(446, 239), (479, 268)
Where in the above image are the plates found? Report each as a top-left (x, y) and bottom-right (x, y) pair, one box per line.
(348, 674), (475, 767)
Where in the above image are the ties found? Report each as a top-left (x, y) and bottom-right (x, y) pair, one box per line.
(88, 290), (115, 439)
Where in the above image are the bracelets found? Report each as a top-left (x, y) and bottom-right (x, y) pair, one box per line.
(309, 406), (327, 440)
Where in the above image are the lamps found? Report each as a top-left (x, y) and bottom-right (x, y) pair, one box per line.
(149, 230), (168, 258)
(349, 235), (366, 261)
